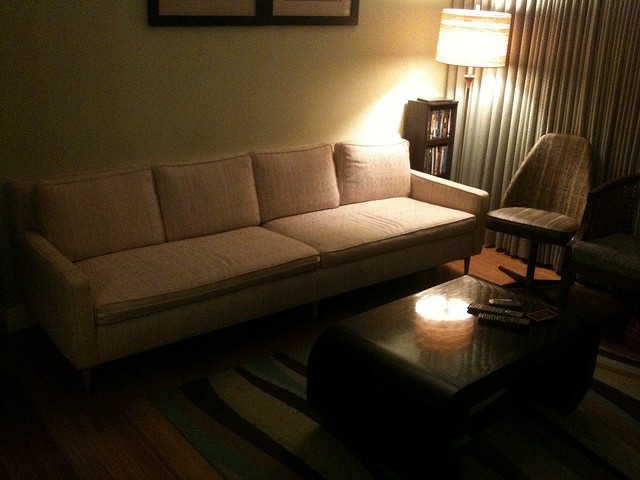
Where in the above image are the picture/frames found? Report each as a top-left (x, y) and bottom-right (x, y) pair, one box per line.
(146, 0), (359, 26)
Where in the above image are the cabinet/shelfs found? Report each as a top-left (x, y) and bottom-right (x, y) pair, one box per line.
(403, 96), (459, 180)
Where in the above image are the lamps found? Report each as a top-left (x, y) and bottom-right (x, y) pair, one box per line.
(435, 8), (512, 183)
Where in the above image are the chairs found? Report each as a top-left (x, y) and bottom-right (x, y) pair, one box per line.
(486, 133), (596, 308)
(561, 174), (640, 305)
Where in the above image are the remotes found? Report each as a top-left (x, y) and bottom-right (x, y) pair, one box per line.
(467, 302), (525, 317)
(488, 298), (520, 307)
(477, 312), (530, 330)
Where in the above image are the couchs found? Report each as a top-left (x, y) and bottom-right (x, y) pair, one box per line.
(2, 137), (489, 400)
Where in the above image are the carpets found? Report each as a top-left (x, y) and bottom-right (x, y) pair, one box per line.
(146, 274), (639, 479)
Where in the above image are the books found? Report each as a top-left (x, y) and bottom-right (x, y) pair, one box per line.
(424, 146), (449, 176)
(427, 109), (450, 141)
